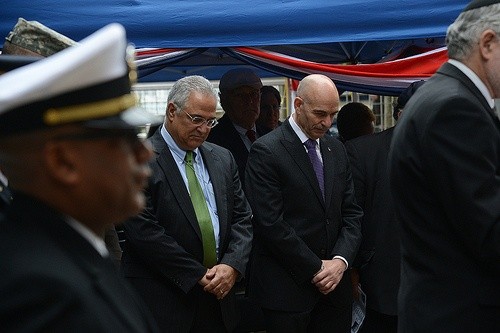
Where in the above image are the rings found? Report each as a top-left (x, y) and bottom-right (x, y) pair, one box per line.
(220, 290), (223, 294)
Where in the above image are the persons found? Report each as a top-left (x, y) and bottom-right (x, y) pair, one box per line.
(0, 17), (77, 75)
(0, 22), (162, 332)
(336, 101), (376, 141)
(257, 85), (282, 136)
(244, 72), (366, 333)
(207, 63), (263, 170)
(121, 74), (252, 333)
(387, 0), (500, 333)
(346, 78), (426, 333)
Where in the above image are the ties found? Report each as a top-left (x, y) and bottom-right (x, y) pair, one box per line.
(246, 130), (257, 144)
(184, 152), (218, 269)
(304, 138), (325, 195)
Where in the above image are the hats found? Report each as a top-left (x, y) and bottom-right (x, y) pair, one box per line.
(1, 23), (163, 133)
(219, 69), (263, 94)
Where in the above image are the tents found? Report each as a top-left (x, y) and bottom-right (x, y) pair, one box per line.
(0, 0), (500, 131)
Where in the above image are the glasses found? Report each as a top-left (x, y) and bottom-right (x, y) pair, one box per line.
(262, 105), (282, 111)
(174, 103), (218, 128)
